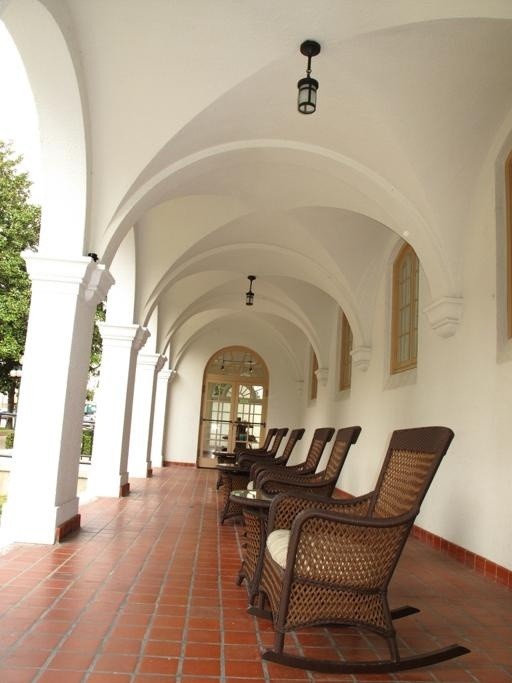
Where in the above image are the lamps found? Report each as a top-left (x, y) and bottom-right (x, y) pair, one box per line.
(246, 275), (256, 305)
(296, 39), (321, 115)
